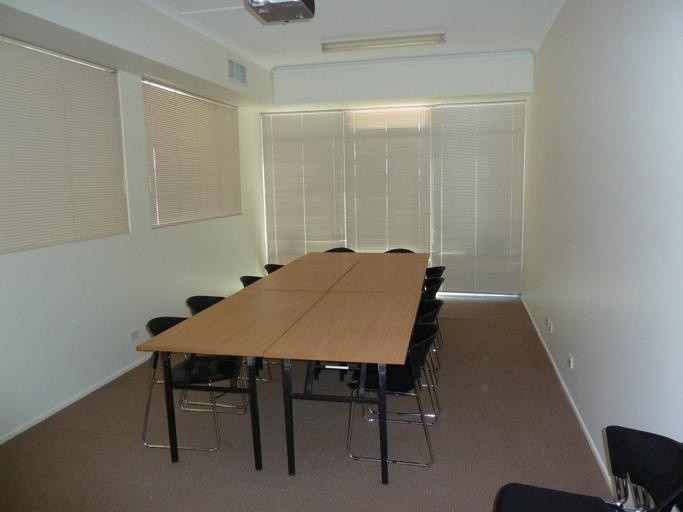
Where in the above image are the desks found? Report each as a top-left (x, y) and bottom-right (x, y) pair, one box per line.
(137, 253), (431, 476)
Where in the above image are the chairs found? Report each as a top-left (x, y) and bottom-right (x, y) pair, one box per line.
(234, 273), (287, 384)
(343, 335), (436, 469)
(178, 293), (249, 417)
(263, 261), (285, 272)
(415, 265), (451, 388)
(492, 420), (683, 512)
(140, 314), (248, 454)
